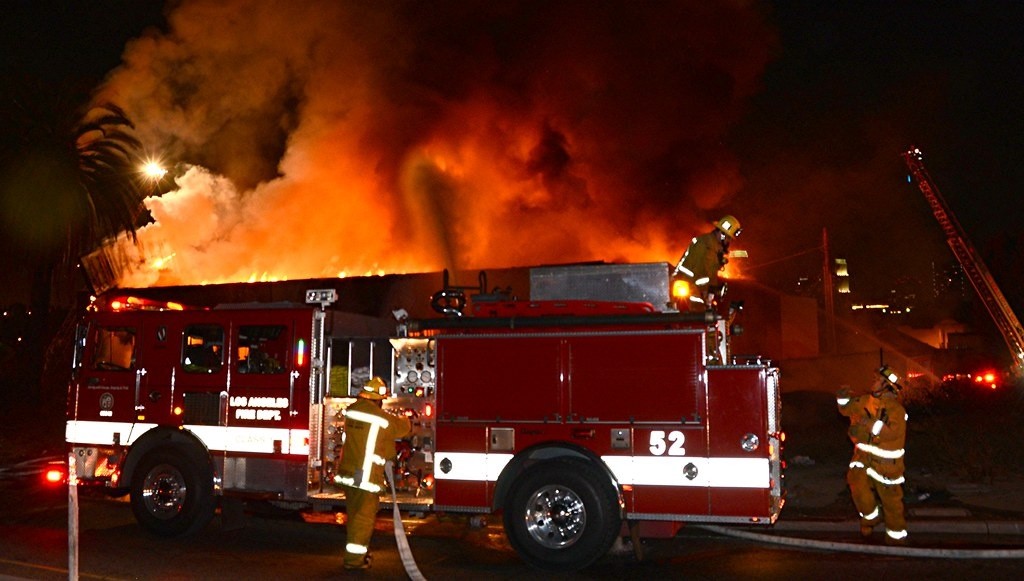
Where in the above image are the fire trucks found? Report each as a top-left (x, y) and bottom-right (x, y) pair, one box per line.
(903, 145), (1024, 377)
(62, 261), (790, 576)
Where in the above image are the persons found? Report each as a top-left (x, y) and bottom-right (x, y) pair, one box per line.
(670, 215), (741, 364)
(832, 363), (909, 544)
(335, 378), (410, 570)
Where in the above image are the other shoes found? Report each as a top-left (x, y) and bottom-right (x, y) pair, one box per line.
(860, 526), (872, 535)
(885, 534), (906, 545)
(344, 554), (373, 568)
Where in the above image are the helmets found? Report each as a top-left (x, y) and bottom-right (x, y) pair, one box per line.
(878, 363), (904, 389)
(359, 377), (389, 399)
(712, 215), (742, 241)
